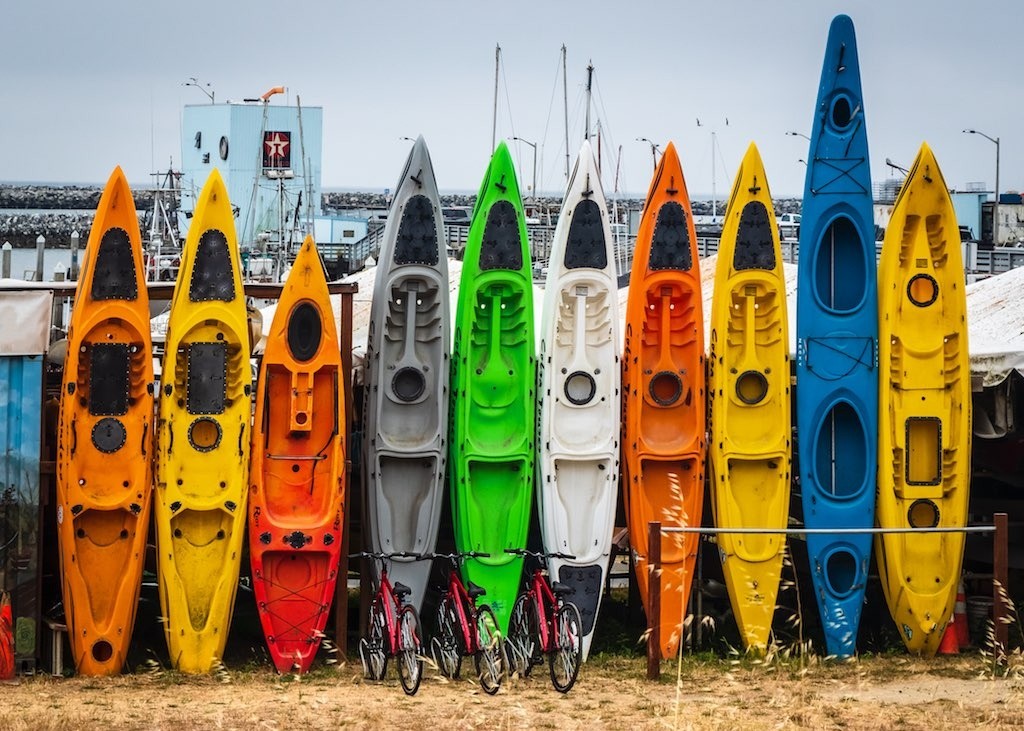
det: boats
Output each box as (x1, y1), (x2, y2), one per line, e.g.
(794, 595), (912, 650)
(450, 137), (536, 654)
(537, 137), (624, 666)
(154, 165), (248, 679)
(795, 12), (880, 664)
(622, 140), (708, 668)
(250, 234), (346, 681)
(54, 163), (156, 676)
(875, 140), (975, 657)
(359, 133), (451, 649)
(708, 139), (792, 662)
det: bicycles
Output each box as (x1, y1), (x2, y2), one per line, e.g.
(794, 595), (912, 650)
(504, 547), (583, 693)
(356, 550), (426, 696)
(416, 549), (506, 695)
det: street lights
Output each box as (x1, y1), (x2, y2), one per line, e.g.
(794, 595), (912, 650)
(180, 83), (214, 105)
(962, 129), (1000, 248)
(513, 137), (537, 199)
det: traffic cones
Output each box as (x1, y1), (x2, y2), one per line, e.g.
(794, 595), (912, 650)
(953, 577), (979, 653)
(938, 612), (963, 657)
